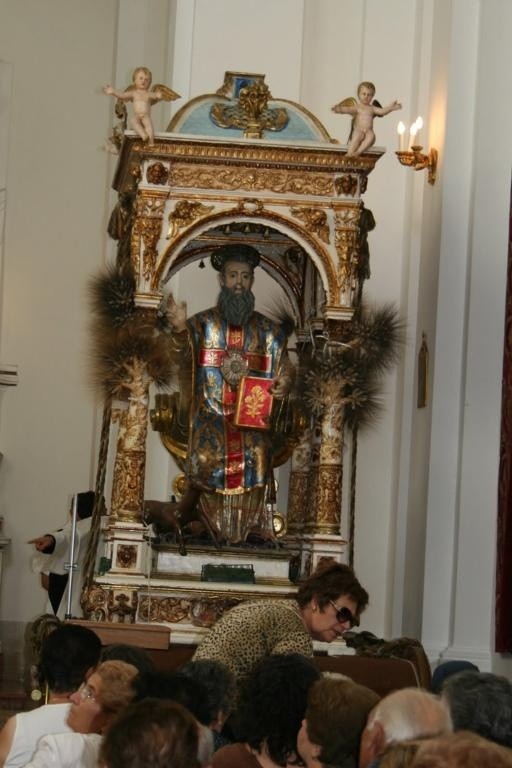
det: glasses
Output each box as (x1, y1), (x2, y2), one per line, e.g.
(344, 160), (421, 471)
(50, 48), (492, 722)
(328, 599), (360, 628)
(78, 683), (97, 703)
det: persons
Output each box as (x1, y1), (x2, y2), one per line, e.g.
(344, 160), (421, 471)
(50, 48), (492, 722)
(164, 244), (311, 548)
(297, 631), (512, 767)
(98, 64), (167, 149)
(28, 492), (109, 616)
(330, 79), (405, 161)
(0, 624), (324, 767)
(191, 561), (371, 752)
